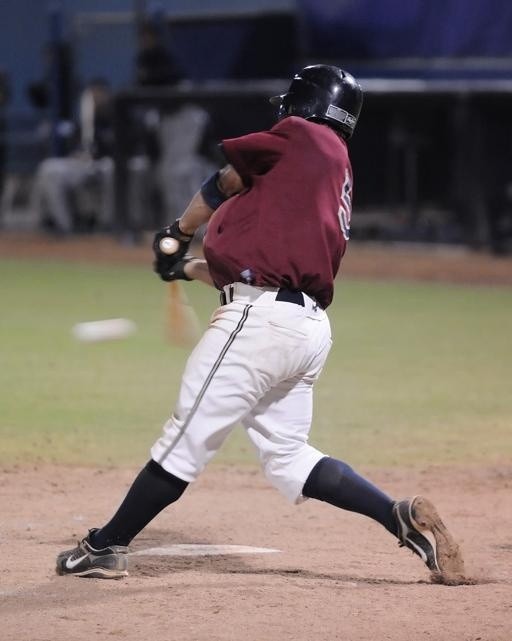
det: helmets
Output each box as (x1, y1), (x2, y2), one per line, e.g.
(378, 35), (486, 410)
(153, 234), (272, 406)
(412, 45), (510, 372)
(269, 64), (363, 141)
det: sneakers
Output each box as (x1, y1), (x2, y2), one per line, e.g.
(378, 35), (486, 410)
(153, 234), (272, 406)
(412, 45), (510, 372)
(56, 527), (128, 578)
(394, 495), (465, 581)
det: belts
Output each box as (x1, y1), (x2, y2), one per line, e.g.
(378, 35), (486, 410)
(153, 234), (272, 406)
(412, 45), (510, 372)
(219, 286), (304, 307)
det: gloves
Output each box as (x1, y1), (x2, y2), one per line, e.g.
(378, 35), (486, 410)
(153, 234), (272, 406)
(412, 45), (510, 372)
(155, 254), (198, 281)
(153, 220), (194, 270)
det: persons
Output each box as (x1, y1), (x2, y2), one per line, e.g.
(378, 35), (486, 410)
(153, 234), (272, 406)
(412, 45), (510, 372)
(55, 64), (466, 587)
